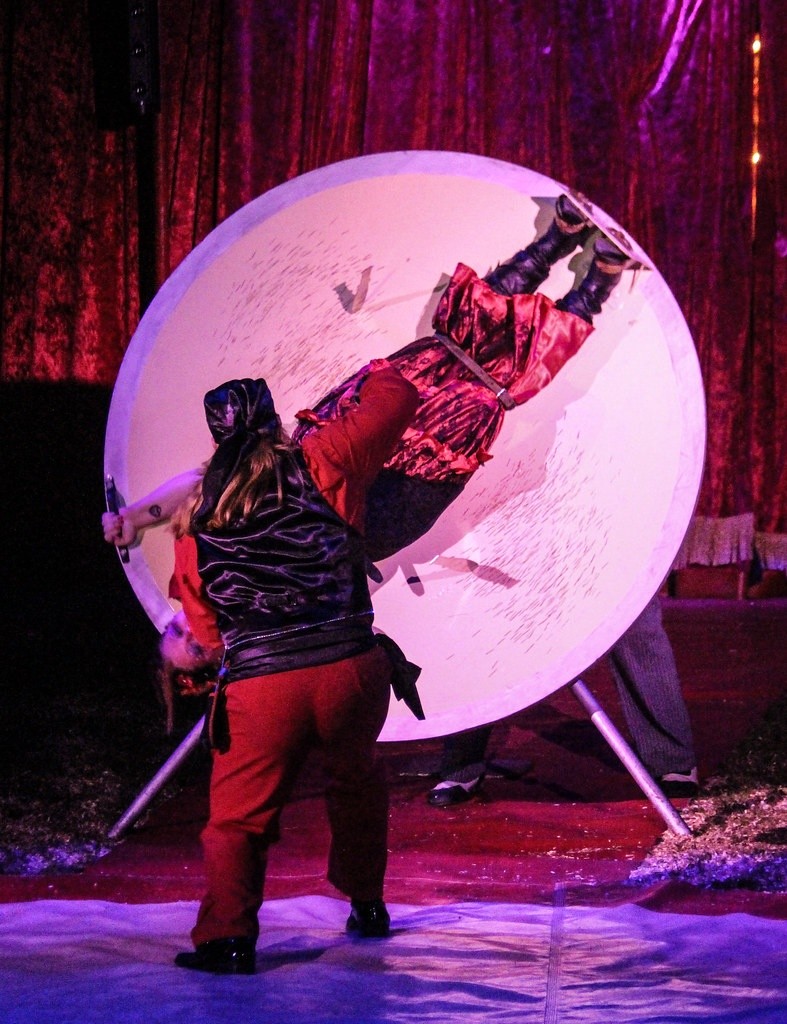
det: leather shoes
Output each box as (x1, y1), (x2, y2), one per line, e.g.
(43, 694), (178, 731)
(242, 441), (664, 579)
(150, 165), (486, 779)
(175, 937), (257, 974)
(346, 898), (390, 937)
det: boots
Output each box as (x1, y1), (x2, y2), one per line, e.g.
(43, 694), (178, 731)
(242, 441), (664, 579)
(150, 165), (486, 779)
(579, 227), (632, 303)
(526, 193), (595, 266)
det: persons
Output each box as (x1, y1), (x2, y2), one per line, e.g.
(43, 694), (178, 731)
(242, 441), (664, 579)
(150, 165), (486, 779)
(100, 192), (638, 733)
(428, 595), (699, 807)
(178, 357), (423, 970)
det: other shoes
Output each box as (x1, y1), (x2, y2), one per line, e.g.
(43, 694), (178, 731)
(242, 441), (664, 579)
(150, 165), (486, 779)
(428, 770), (484, 806)
(661, 767), (699, 797)
